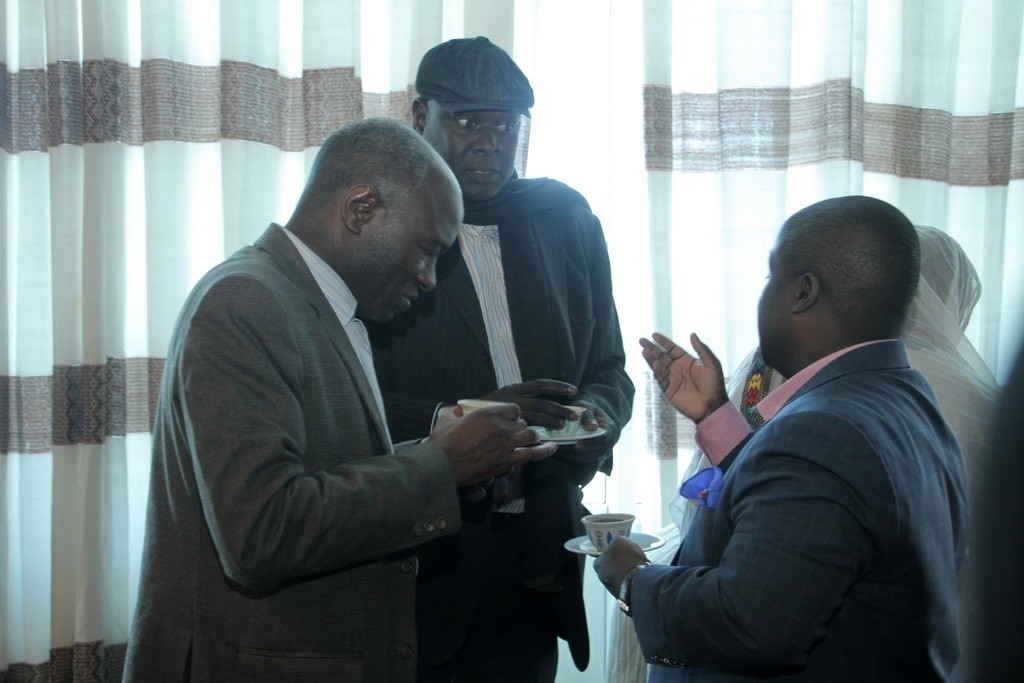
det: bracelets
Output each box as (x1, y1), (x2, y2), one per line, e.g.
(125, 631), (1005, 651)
(616, 562), (650, 616)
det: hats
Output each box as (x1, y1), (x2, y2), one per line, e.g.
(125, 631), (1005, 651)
(415, 36), (534, 118)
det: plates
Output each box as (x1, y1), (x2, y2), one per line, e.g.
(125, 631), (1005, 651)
(564, 533), (666, 557)
(526, 425), (606, 445)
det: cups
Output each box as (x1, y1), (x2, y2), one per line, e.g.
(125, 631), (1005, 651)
(581, 514), (635, 551)
(458, 400), (505, 417)
(545, 406), (587, 437)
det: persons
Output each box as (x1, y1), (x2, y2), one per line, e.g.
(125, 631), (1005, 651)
(141, 117), (558, 682)
(358, 32), (636, 683)
(589, 195), (997, 682)
